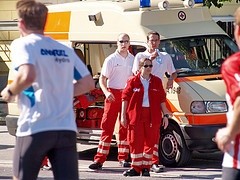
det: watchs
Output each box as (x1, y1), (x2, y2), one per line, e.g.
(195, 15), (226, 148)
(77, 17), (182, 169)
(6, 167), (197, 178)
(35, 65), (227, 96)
(163, 114), (169, 117)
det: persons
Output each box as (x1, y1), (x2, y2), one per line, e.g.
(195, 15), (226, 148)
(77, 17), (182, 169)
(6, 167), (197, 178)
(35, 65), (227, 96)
(183, 46), (209, 70)
(120, 58), (169, 176)
(214, 6), (240, 180)
(132, 31), (178, 173)
(40, 154), (50, 168)
(0, 0), (96, 180)
(88, 33), (136, 170)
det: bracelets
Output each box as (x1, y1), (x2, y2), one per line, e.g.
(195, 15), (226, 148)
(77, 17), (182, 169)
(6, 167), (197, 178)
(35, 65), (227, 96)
(7, 87), (15, 97)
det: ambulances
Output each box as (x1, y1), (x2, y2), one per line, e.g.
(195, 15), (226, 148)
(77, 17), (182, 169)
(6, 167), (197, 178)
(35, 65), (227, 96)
(5, 0), (240, 168)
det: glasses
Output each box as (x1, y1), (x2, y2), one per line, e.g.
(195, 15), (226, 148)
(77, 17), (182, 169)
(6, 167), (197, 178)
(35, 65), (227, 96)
(118, 40), (129, 43)
(143, 65), (153, 67)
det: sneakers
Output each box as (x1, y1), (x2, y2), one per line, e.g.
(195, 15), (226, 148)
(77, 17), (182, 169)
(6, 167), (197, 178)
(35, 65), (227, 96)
(150, 164), (161, 172)
(118, 160), (131, 168)
(142, 168), (150, 176)
(89, 161), (102, 169)
(41, 166), (51, 170)
(123, 167), (140, 176)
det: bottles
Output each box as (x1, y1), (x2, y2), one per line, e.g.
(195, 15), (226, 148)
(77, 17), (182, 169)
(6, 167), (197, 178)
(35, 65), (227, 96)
(155, 48), (163, 65)
